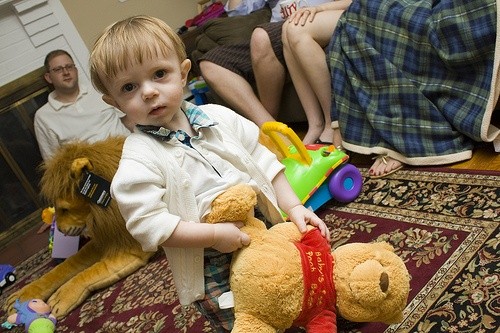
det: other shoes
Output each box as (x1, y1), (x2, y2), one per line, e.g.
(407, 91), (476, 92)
(314, 138), (332, 145)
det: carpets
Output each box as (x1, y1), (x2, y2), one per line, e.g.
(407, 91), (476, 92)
(0, 157), (500, 333)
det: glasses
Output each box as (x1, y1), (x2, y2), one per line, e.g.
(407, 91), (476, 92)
(48, 64), (75, 73)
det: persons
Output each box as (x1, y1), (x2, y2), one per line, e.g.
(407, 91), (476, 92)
(90, 14), (331, 332)
(33, 0), (500, 179)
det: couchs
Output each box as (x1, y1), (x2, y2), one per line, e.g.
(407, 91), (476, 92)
(191, 6), (308, 124)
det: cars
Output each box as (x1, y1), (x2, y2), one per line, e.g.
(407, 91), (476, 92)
(260, 120), (363, 224)
(0, 264), (17, 291)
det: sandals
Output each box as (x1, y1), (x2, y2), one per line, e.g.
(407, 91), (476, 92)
(369, 153), (405, 178)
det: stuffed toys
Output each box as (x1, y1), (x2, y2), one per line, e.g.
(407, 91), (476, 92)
(206, 184), (411, 333)
(0, 135), (156, 333)
